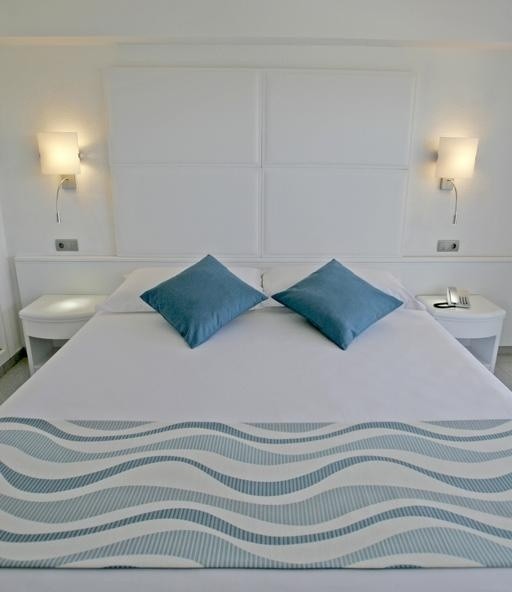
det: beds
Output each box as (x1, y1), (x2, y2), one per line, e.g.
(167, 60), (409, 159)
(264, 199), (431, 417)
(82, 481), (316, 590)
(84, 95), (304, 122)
(1, 267), (512, 592)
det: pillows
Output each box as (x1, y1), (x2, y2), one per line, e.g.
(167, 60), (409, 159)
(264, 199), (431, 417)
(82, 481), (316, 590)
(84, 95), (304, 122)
(139, 254), (269, 349)
(270, 258), (404, 351)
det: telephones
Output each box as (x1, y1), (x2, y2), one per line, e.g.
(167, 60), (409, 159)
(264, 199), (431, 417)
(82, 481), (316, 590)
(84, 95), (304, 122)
(447, 286), (472, 308)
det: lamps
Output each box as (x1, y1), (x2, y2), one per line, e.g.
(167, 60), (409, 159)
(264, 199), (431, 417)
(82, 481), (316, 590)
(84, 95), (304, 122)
(38, 131), (85, 223)
(437, 135), (478, 224)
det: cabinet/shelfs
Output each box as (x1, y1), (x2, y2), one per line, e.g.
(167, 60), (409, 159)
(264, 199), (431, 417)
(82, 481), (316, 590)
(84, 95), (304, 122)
(18, 293), (109, 377)
(417, 295), (505, 374)
